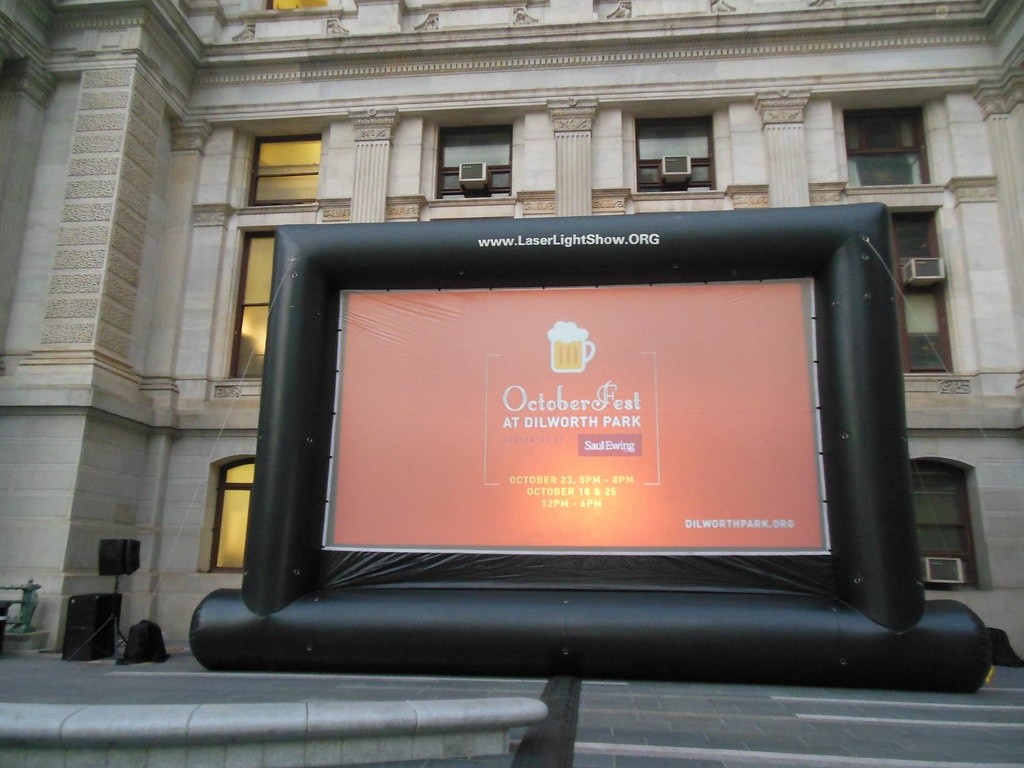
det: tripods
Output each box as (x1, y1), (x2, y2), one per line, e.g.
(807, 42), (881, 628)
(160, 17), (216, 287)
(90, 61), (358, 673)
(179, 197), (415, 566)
(68, 574), (127, 664)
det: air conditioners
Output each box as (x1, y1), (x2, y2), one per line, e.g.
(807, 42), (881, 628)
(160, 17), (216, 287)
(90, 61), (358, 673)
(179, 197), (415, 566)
(923, 557), (965, 584)
(659, 155), (692, 184)
(902, 258), (945, 286)
(459, 162), (493, 190)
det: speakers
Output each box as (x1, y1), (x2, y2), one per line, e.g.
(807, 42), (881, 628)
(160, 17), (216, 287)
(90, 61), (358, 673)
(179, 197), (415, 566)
(98, 539), (140, 577)
(63, 592), (123, 662)
